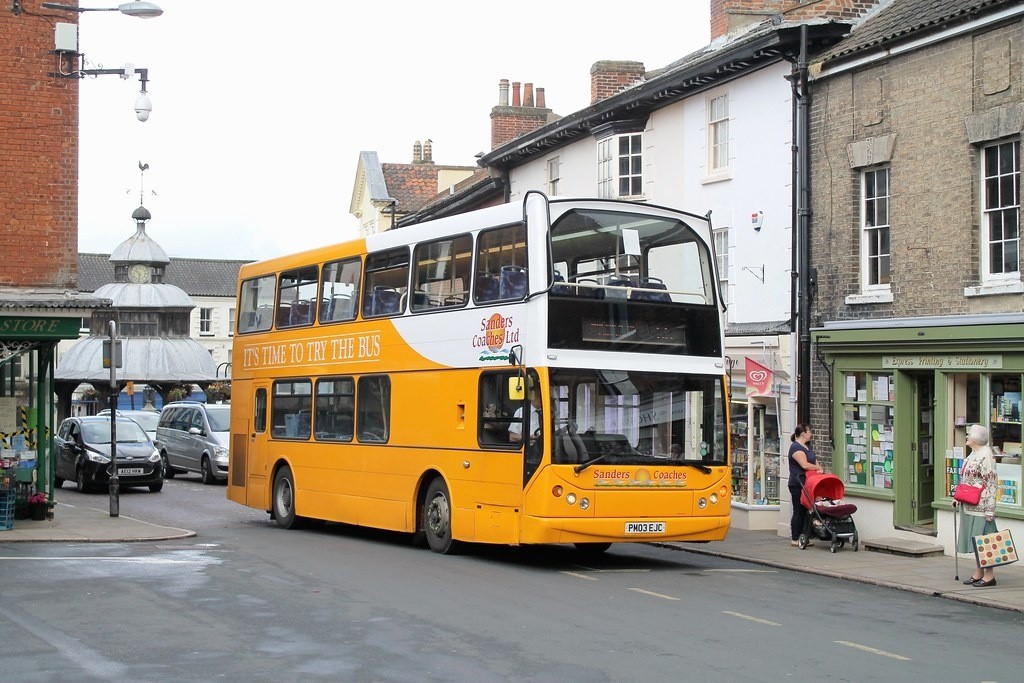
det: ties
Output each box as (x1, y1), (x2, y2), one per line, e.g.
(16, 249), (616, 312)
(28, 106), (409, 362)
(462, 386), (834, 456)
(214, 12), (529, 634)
(535, 410), (543, 432)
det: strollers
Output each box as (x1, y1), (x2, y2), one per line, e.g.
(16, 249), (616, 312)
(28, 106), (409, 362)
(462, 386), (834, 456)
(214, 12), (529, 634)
(794, 471), (860, 554)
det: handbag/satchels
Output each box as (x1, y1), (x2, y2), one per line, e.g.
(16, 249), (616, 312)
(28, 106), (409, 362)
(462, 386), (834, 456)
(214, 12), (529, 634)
(972, 529), (1019, 569)
(954, 484), (982, 506)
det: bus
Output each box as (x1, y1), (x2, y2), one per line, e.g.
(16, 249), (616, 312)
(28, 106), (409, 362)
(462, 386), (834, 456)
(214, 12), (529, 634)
(226, 189), (731, 555)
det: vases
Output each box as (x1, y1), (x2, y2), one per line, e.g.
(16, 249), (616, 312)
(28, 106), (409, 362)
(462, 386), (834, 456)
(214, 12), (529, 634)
(30, 503), (47, 520)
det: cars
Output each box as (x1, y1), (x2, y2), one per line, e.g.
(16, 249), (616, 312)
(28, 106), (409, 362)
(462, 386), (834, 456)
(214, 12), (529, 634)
(46, 416), (164, 492)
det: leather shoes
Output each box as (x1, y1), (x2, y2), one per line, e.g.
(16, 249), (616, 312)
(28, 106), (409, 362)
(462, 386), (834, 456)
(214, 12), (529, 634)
(963, 577), (981, 584)
(972, 576), (997, 587)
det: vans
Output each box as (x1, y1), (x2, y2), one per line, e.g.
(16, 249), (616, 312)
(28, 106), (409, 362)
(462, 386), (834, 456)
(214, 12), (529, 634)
(153, 400), (232, 484)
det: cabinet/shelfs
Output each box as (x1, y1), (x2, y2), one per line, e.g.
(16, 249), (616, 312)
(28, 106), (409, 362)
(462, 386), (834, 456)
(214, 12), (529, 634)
(0, 488), (16, 531)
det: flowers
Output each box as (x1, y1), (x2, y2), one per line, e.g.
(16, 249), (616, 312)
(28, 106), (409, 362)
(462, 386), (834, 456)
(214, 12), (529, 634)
(28, 492), (48, 503)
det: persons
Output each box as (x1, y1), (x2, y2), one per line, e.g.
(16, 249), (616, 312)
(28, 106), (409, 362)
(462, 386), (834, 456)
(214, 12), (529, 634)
(506, 383), (559, 445)
(950, 422), (1001, 588)
(787, 423), (826, 550)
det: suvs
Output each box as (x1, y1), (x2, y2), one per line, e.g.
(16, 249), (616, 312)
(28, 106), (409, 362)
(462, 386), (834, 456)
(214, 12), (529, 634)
(97, 407), (163, 447)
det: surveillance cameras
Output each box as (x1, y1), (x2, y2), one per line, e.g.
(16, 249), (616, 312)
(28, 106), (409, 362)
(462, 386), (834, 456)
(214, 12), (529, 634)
(134, 91), (152, 123)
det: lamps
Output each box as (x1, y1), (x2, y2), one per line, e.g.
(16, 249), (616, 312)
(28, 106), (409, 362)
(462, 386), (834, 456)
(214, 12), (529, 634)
(42, 0), (163, 20)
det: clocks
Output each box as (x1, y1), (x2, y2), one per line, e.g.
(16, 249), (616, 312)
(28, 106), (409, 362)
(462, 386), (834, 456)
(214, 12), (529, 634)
(128, 264), (150, 284)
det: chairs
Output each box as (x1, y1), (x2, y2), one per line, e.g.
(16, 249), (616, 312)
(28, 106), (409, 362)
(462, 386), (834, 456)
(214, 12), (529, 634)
(640, 276), (672, 304)
(270, 406), (389, 442)
(548, 270), (567, 295)
(577, 278), (602, 298)
(278, 302), (293, 326)
(372, 285), (406, 315)
(475, 271), (500, 303)
(326, 293), (353, 321)
(500, 266), (528, 299)
(350, 290), (375, 317)
(288, 300), (309, 325)
(607, 275), (639, 300)
(308, 296), (331, 323)
(255, 303), (274, 329)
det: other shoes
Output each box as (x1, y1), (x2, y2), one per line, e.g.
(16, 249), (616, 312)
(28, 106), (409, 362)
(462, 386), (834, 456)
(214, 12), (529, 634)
(791, 539), (814, 547)
